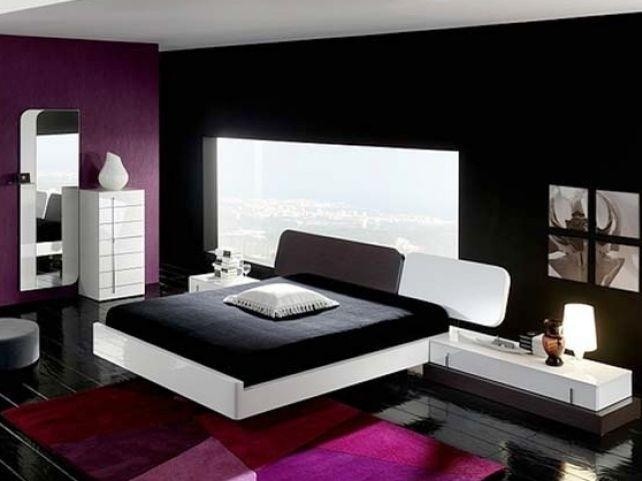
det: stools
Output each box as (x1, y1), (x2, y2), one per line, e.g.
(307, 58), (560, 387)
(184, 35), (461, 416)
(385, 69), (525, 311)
(0, 318), (40, 371)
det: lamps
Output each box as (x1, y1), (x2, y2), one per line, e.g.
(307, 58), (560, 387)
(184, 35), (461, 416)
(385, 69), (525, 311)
(561, 303), (597, 367)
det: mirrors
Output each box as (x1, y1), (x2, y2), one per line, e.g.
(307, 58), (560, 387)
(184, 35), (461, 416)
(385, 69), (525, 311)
(20, 109), (79, 292)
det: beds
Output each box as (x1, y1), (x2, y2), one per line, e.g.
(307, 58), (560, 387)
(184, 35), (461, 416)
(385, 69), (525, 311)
(92, 228), (512, 421)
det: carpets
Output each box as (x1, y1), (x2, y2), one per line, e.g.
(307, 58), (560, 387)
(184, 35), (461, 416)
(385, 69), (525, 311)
(1, 378), (511, 481)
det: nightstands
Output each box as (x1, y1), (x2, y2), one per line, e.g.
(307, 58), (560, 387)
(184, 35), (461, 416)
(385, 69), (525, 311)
(422, 329), (641, 448)
(188, 271), (260, 293)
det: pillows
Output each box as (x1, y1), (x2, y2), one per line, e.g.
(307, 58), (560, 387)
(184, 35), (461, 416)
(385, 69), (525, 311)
(223, 283), (340, 318)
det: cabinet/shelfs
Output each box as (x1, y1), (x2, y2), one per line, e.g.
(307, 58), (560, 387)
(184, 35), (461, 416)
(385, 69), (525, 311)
(78, 188), (146, 302)
